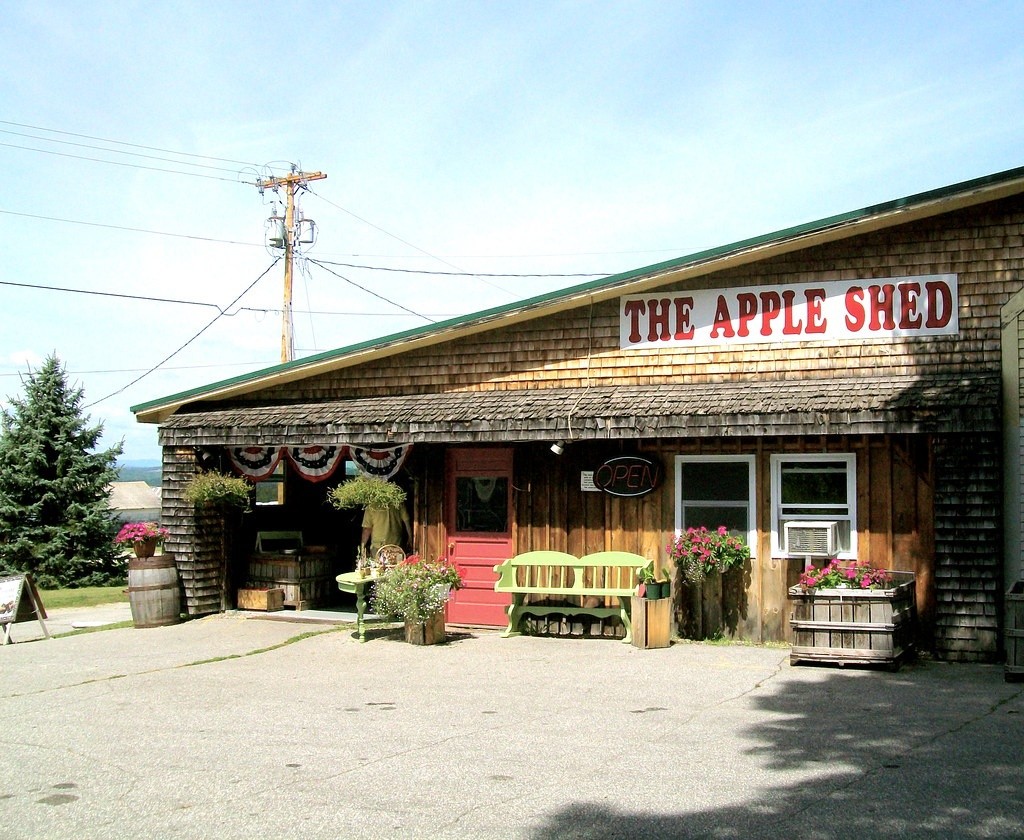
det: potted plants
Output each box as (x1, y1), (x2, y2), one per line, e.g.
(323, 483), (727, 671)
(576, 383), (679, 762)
(660, 568), (672, 598)
(179, 466), (256, 517)
(636, 560), (654, 599)
(642, 567), (661, 600)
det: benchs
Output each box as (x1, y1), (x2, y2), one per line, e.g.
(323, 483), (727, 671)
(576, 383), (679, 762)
(493, 550), (654, 644)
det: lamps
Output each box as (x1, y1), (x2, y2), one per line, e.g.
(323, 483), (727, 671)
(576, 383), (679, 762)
(550, 440), (565, 455)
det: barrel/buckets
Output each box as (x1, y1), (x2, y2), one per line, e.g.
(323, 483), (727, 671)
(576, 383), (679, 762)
(128, 554), (180, 628)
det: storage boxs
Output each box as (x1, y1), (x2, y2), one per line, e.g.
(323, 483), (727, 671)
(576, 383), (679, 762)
(237, 586), (285, 613)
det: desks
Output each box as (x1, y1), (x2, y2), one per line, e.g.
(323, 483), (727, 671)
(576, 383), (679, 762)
(336, 571), (391, 641)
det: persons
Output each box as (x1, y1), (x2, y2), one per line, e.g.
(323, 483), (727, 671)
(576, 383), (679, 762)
(360, 498), (412, 559)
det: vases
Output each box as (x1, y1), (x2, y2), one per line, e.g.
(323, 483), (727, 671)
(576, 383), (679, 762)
(788, 568), (917, 671)
(430, 582), (453, 601)
(135, 542), (157, 558)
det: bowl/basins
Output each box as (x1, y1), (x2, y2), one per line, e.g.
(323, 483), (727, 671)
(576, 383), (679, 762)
(279, 548), (297, 555)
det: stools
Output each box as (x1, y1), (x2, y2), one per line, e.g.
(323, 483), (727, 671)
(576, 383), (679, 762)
(630, 595), (673, 649)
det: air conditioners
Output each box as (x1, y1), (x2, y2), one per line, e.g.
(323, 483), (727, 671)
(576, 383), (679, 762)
(783, 521), (842, 556)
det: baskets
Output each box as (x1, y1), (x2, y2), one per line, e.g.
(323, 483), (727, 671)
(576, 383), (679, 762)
(375, 544), (406, 570)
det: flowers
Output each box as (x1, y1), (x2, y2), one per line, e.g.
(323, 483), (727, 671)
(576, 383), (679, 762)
(367, 554), (468, 626)
(799, 556), (894, 594)
(665, 526), (749, 585)
(113, 522), (171, 544)
